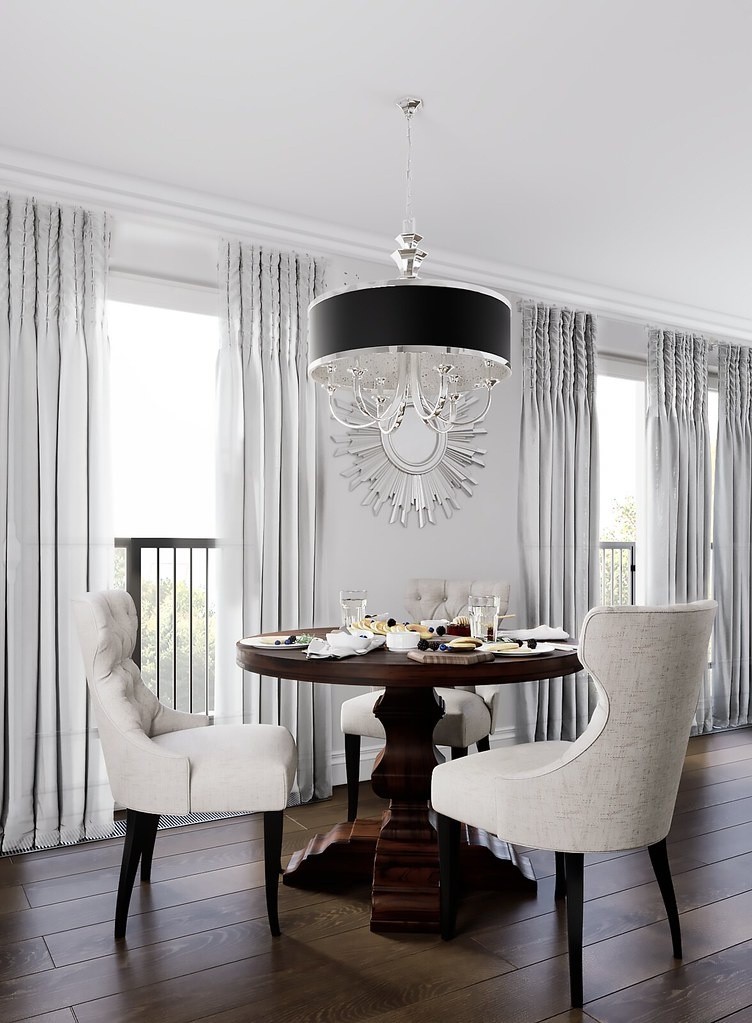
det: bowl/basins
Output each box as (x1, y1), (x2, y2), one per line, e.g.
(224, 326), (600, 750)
(421, 620), (447, 636)
(326, 630), (374, 650)
(386, 632), (420, 651)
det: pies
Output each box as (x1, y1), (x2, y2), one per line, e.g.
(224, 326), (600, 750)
(444, 637), (484, 651)
(349, 618), (433, 639)
(486, 643), (531, 652)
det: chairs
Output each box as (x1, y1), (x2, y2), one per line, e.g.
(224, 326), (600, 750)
(430, 596), (718, 1007)
(73, 588), (297, 941)
(339, 578), (512, 820)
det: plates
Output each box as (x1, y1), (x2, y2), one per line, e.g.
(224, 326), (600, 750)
(240, 636), (323, 649)
(475, 642), (554, 657)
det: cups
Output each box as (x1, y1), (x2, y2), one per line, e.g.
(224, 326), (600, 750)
(468, 595), (499, 643)
(340, 590), (367, 630)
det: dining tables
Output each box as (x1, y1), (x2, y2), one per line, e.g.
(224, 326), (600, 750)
(234, 627), (583, 934)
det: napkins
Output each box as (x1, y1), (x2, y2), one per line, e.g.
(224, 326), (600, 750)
(497, 625), (570, 640)
(302, 634), (386, 658)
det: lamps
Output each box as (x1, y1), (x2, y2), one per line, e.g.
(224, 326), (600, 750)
(306, 96), (513, 434)
